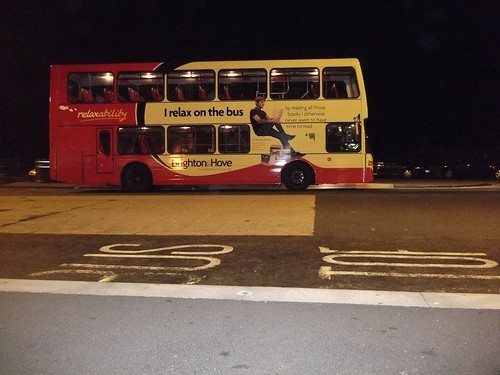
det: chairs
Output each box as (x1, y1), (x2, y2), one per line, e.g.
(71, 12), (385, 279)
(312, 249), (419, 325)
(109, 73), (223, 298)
(66, 81), (340, 154)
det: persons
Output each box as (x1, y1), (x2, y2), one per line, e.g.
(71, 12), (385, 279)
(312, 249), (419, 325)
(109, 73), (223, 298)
(328, 127), (349, 152)
(252, 98), (308, 158)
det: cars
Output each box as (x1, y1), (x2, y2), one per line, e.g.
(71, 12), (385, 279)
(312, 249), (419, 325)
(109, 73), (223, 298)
(371, 151), (433, 180)
(5, 157), (48, 179)
(433, 150), (500, 179)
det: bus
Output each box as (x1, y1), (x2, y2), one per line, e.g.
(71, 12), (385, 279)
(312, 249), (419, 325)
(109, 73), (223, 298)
(47, 57), (376, 194)
